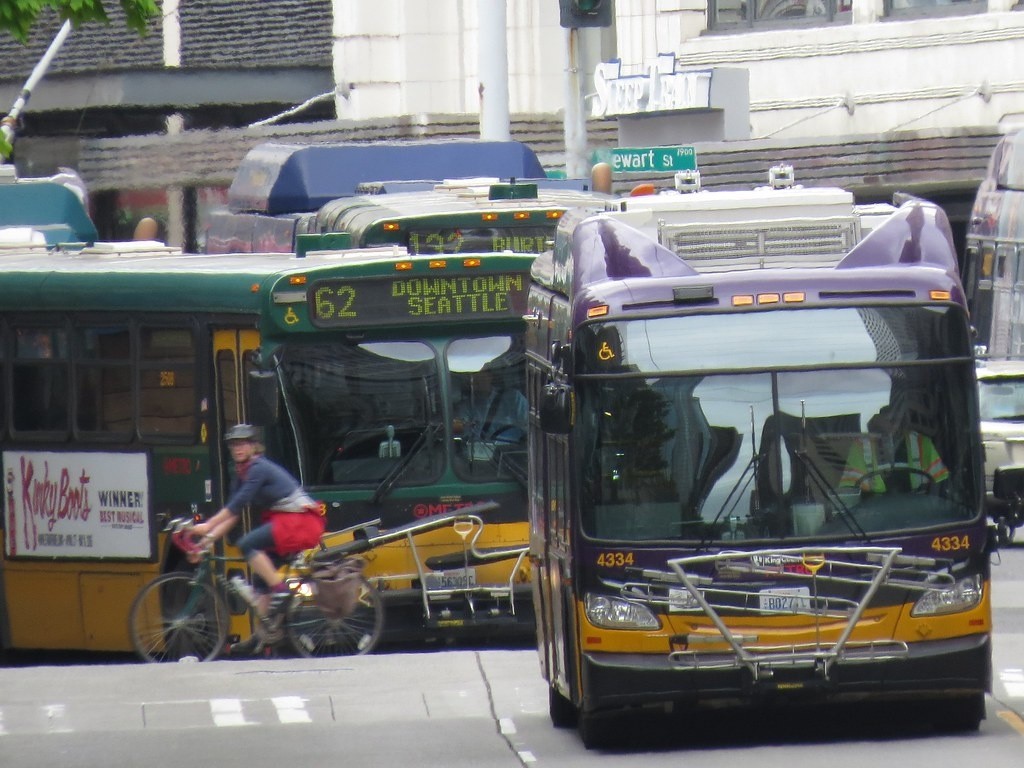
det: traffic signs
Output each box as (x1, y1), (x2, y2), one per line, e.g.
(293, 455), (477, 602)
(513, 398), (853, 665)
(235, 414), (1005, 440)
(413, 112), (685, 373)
(586, 146), (697, 177)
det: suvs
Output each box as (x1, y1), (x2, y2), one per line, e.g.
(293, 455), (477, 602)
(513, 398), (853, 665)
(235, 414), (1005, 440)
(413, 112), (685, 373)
(976, 360), (1024, 533)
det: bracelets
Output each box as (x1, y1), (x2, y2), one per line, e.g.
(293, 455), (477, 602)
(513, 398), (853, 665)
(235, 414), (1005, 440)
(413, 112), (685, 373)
(206, 533), (215, 539)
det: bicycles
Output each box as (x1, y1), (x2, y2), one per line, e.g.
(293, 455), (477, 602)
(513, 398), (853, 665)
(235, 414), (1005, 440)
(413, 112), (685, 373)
(127, 514), (382, 660)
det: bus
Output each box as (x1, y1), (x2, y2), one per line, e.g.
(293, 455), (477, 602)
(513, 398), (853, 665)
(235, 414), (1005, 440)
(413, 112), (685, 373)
(967, 123), (1024, 360)
(1, 241), (546, 660)
(298, 179), (618, 252)
(202, 141), (547, 249)
(523, 184), (989, 749)
(1, 166), (98, 242)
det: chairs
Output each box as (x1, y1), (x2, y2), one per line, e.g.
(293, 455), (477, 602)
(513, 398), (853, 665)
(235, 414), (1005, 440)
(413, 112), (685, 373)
(808, 431), (868, 504)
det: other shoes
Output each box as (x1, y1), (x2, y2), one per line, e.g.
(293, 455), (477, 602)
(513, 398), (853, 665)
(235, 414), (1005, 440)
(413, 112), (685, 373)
(268, 589), (294, 615)
(230, 634), (258, 653)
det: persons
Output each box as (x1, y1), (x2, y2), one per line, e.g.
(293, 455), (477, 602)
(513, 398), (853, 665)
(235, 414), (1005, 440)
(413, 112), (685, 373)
(837, 408), (950, 506)
(192, 424), (324, 622)
(458, 371), (528, 444)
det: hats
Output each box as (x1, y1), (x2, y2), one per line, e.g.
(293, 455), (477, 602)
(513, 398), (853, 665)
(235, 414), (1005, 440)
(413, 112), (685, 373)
(223, 424), (260, 441)
